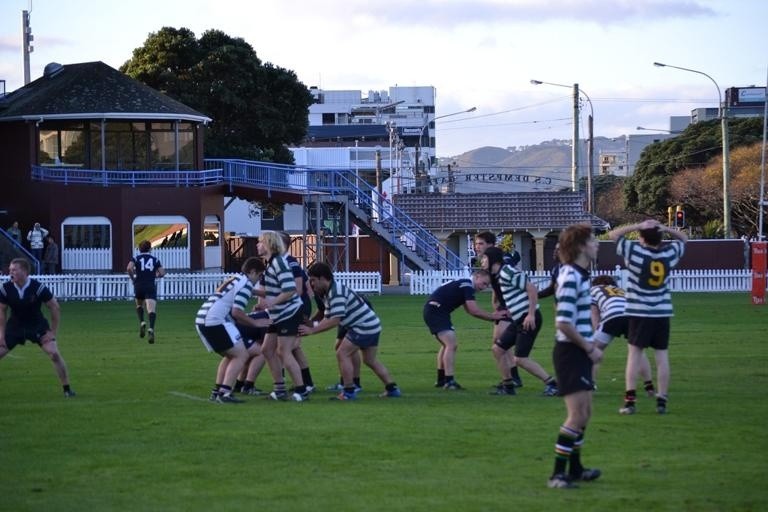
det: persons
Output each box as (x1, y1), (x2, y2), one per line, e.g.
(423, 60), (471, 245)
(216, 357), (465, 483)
(537, 240), (563, 303)
(195, 230), (402, 404)
(127, 240), (165, 344)
(5, 223), (59, 274)
(547, 223), (604, 487)
(425, 269), (511, 390)
(608, 220), (688, 416)
(468, 232), (558, 396)
(590, 276), (656, 397)
(0, 258), (76, 398)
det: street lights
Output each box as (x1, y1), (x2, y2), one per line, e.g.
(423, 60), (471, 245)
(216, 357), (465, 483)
(635, 125), (683, 136)
(418, 107), (476, 169)
(653, 61), (733, 238)
(529, 79), (596, 217)
(386, 119), (407, 218)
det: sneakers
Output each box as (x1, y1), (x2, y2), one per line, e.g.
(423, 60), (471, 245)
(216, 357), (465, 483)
(325, 383), (401, 400)
(547, 468), (602, 490)
(147, 327), (154, 344)
(435, 376), (466, 392)
(63, 390), (77, 398)
(488, 376), (562, 397)
(209, 382), (317, 403)
(618, 389), (669, 415)
(139, 321), (147, 337)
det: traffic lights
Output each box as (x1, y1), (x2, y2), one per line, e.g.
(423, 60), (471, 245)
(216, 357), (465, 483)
(675, 210), (685, 228)
